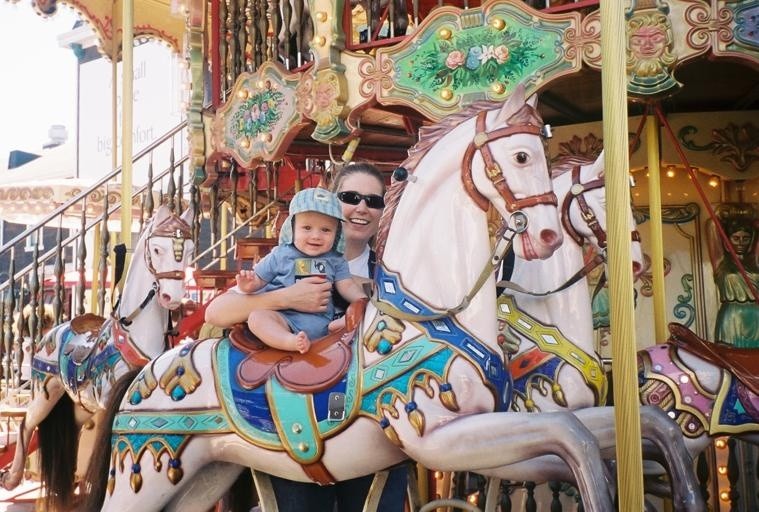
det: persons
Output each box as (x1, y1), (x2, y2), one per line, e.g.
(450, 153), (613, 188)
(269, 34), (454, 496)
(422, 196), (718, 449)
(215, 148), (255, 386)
(237, 186), (369, 353)
(205, 163), (409, 512)
(703, 202), (759, 350)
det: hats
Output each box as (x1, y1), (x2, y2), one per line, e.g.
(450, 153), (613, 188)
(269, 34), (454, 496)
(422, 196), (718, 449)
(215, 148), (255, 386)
(280, 187), (350, 254)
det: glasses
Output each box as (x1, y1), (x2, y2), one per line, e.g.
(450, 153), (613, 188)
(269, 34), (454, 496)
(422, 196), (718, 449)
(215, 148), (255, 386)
(336, 191), (385, 209)
